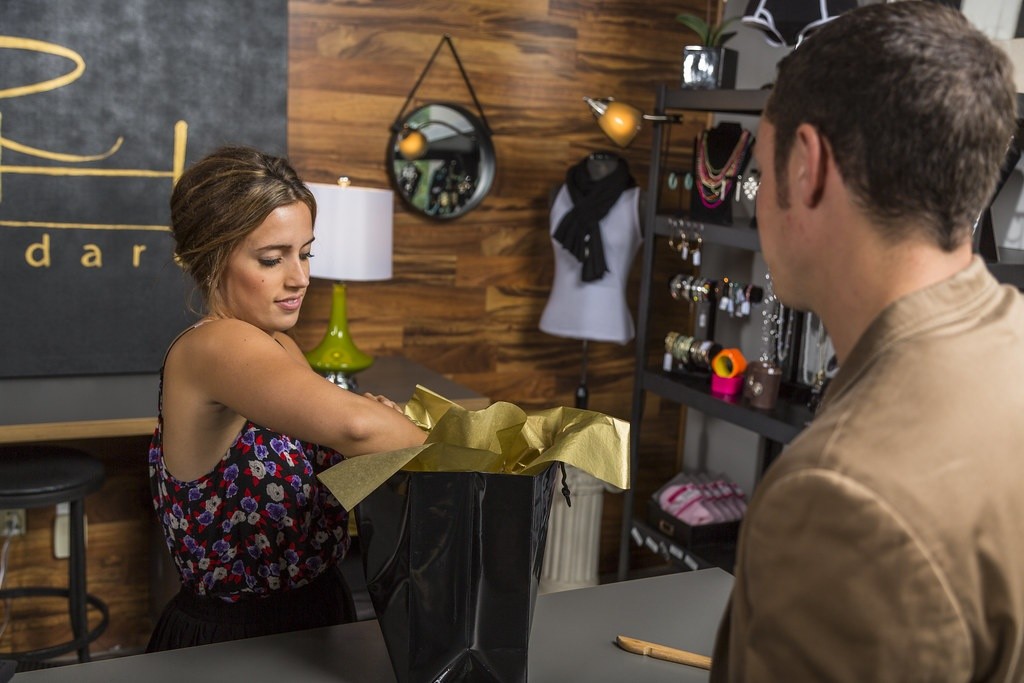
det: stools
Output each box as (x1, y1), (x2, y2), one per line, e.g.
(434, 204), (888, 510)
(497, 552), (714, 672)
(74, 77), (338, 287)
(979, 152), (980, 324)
(0, 446), (113, 677)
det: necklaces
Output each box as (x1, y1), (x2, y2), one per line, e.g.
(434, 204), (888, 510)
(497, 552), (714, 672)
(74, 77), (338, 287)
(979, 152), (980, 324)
(693, 126), (757, 209)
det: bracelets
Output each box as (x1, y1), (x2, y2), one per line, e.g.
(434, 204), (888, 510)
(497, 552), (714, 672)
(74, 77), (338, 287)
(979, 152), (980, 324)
(662, 329), (781, 411)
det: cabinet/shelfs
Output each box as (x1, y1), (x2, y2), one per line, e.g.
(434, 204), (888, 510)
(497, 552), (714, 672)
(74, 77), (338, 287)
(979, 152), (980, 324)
(614, 78), (1023, 592)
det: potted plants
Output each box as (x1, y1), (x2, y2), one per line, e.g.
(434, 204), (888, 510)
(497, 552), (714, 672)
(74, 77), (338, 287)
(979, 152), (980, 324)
(677, 12), (743, 90)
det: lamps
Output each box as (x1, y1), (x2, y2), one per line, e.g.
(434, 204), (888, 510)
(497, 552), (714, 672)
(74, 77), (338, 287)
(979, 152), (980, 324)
(301, 176), (395, 372)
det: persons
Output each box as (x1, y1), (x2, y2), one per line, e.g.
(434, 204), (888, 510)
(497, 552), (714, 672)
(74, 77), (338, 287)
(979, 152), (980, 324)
(146, 144), (440, 658)
(705, 0), (1023, 680)
(535, 151), (652, 347)
